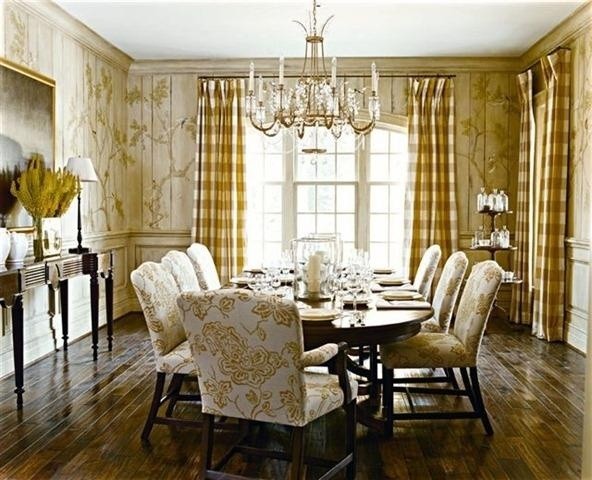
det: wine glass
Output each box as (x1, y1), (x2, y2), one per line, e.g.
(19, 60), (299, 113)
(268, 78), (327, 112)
(328, 249), (375, 319)
(248, 250), (293, 297)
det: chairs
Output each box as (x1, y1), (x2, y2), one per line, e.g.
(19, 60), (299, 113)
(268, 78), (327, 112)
(161, 249), (201, 394)
(130, 261), (253, 442)
(371, 250), (470, 399)
(378, 260), (504, 437)
(177, 288), (359, 480)
(360, 245), (442, 376)
(186, 242), (222, 290)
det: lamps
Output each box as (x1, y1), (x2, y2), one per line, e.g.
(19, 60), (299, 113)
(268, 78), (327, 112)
(66, 156), (98, 254)
(239, 0), (380, 167)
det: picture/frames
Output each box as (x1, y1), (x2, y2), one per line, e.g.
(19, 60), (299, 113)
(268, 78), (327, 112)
(0, 57), (58, 234)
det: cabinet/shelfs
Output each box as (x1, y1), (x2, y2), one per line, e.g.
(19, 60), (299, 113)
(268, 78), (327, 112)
(466, 210), (524, 335)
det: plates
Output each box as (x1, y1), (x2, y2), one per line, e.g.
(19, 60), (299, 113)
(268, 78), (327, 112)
(244, 268), (265, 274)
(299, 308), (339, 323)
(230, 277), (252, 285)
(374, 267), (421, 301)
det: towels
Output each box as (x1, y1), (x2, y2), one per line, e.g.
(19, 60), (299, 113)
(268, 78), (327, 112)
(376, 300), (431, 309)
(372, 273), (393, 279)
(371, 284), (417, 292)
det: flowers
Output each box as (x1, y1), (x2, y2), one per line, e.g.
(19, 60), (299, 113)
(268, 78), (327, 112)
(8, 153), (77, 217)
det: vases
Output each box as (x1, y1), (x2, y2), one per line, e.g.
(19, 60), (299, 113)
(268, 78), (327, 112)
(32, 215), (43, 258)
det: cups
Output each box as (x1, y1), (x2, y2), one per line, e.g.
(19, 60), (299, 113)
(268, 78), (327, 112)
(504, 270), (514, 283)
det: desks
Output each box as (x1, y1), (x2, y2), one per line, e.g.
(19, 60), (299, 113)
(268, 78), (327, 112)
(0, 249), (115, 412)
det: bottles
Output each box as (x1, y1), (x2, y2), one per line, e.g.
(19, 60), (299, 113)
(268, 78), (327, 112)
(476, 187), (508, 211)
(471, 225), (510, 249)
